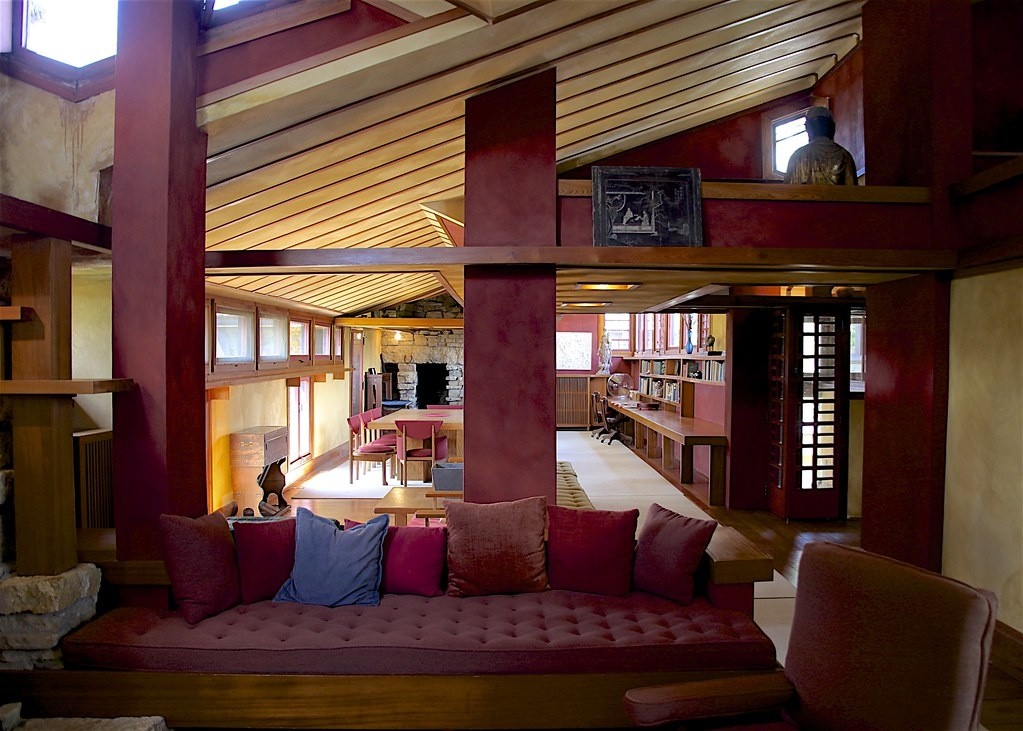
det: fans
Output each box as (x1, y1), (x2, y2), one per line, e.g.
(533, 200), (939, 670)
(607, 373), (635, 397)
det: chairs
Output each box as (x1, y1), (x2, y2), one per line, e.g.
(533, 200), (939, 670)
(590, 391), (633, 446)
(347, 404), (464, 487)
(623, 542), (996, 731)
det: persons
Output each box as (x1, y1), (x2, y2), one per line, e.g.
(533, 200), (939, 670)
(785, 106), (858, 188)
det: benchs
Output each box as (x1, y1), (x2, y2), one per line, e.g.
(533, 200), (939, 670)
(56, 523), (777, 731)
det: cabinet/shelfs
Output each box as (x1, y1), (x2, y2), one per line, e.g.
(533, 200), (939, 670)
(231, 426), (290, 521)
(623, 355), (727, 417)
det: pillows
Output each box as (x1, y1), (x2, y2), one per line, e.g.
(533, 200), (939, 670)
(158, 496), (718, 605)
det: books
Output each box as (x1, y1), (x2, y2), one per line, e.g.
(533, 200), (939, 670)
(639, 360), (725, 404)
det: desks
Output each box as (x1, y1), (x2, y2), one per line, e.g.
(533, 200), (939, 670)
(374, 488), (444, 527)
(606, 395), (727, 505)
(367, 409), (464, 480)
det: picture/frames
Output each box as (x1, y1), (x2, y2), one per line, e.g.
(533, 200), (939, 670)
(592, 166), (704, 248)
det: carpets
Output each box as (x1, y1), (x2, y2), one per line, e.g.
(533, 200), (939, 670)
(556, 459), (595, 508)
(292, 457), (432, 499)
